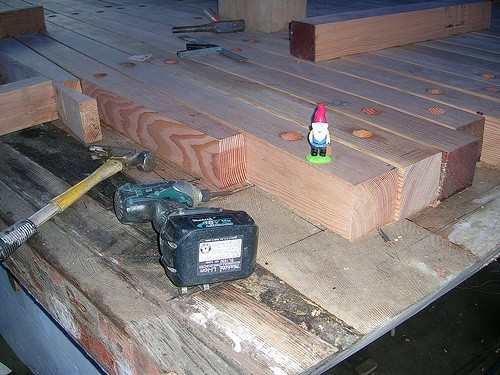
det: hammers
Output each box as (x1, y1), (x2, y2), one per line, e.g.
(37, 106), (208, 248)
(0, 145), (157, 266)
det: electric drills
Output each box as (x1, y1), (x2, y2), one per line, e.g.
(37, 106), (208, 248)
(113, 182), (235, 226)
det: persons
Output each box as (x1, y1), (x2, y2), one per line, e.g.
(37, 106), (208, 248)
(308, 103), (331, 157)
